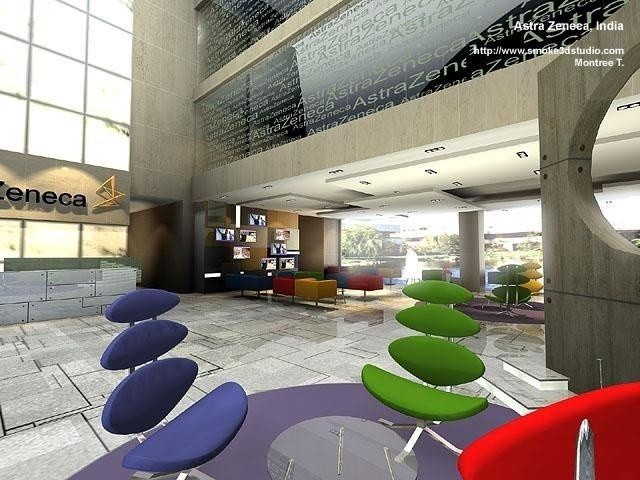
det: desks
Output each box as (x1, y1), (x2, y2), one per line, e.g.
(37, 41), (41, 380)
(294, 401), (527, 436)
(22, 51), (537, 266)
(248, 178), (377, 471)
(264, 415), (420, 479)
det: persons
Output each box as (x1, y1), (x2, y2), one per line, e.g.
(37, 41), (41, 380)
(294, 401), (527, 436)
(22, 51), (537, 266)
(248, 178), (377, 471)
(262, 260), (276, 270)
(234, 247), (250, 259)
(276, 231), (290, 240)
(274, 244), (286, 254)
(218, 229), (234, 240)
(240, 232), (256, 242)
(251, 214), (265, 225)
(282, 259), (294, 268)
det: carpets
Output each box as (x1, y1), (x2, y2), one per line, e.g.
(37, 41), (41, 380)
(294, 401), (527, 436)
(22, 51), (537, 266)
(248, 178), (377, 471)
(65, 384), (522, 480)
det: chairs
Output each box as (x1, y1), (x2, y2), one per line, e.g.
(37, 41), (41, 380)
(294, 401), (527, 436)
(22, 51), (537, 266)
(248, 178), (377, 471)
(362, 262), (640, 479)
(225, 270), (384, 307)
(100, 289), (249, 479)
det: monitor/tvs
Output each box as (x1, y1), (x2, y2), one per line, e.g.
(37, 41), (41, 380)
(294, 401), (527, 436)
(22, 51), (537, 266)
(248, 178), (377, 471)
(279, 257), (295, 270)
(232, 246), (251, 260)
(260, 258), (277, 270)
(248, 213), (267, 227)
(274, 228), (291, 241)
(239, 230), (257, 242)
(270, 242), (287, 256)
(215, 228), (235, 242)
